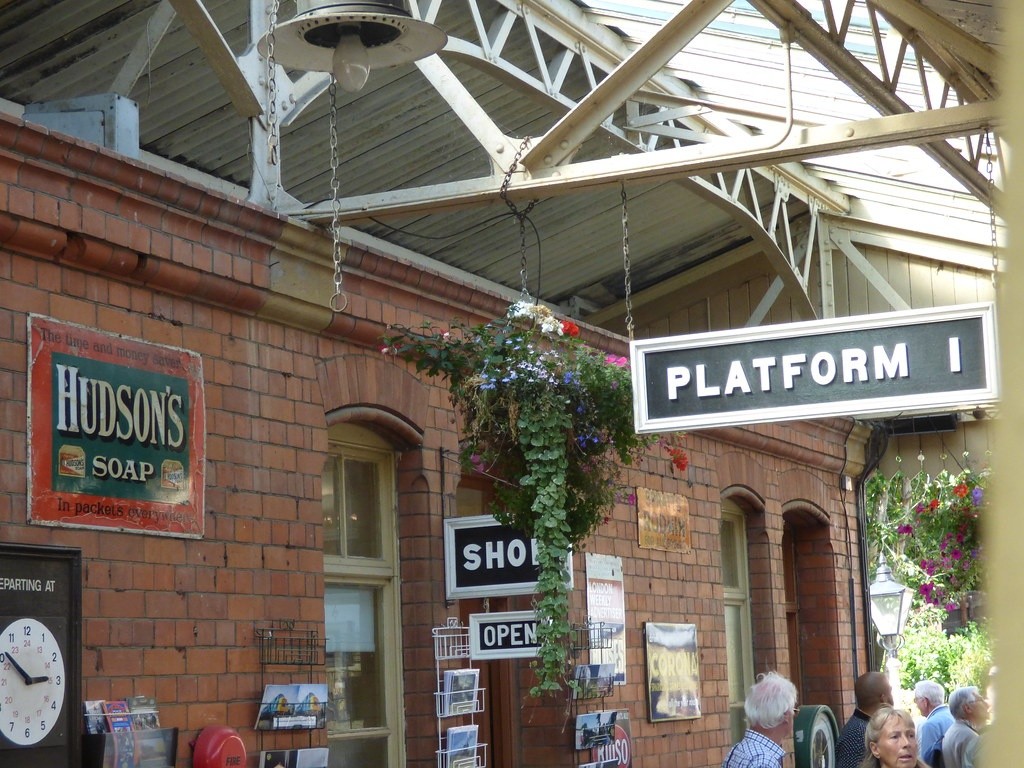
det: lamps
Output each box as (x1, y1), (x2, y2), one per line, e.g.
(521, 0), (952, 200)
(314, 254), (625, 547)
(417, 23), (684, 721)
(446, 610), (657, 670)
(256, 0), (448, 93)
(868, 552), (918, 657)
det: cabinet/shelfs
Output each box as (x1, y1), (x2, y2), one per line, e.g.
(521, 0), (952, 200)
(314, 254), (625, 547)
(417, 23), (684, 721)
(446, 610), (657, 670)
(431, 616), (490, 768)
(568, 615), (626, 768)
(255, 619), (330, 768)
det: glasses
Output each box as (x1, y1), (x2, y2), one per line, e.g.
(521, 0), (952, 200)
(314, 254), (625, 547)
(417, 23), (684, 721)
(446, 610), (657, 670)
(968, 697), (984, 703)
(790, 706), (800, 718)
(913, 697), (920, 703)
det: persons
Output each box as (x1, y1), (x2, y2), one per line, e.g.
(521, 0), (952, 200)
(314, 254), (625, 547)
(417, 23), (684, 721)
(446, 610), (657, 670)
(722, 671), (798, 768)
(837, 667), (997, 768)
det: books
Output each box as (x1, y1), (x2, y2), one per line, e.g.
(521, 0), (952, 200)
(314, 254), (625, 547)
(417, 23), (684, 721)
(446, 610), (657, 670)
(443, 668), (480, 768)
(259, 748), (329, 768)
(256, 684), (328, 730)
(574, 663), (618, 768)
(83, 695), (167, 768)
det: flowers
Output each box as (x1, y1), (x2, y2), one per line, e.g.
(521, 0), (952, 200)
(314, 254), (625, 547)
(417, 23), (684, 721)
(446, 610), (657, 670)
(377, 300), (690, 705)
(871, 485), (987, 611)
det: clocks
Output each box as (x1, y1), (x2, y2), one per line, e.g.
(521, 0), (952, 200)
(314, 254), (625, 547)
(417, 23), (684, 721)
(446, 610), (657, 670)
(793, 704), (839, 768)
(0, 543), (83, 768)
